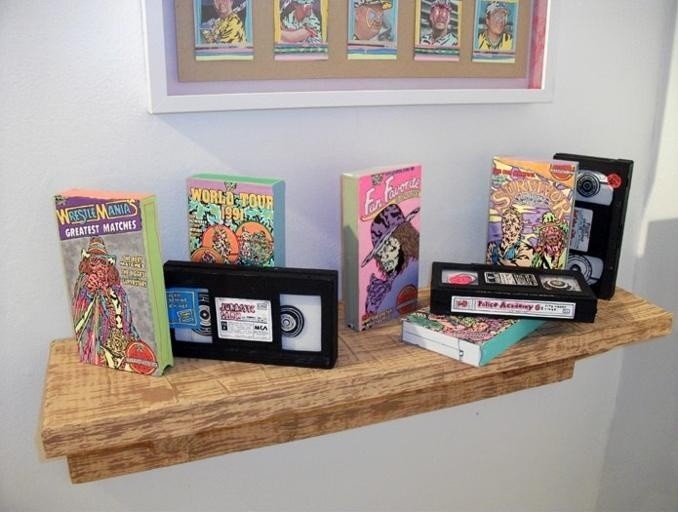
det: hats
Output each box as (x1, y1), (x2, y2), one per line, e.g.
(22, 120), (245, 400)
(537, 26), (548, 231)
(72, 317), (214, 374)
(293, 0), (314, 4)
(80, 236), (116, 267)
(360, 204), (420, 267)
(430, 0), (452, 10)
(539, 211), (558, 229)
(485, 1), (507, 14)
(354, 0), (393, 10)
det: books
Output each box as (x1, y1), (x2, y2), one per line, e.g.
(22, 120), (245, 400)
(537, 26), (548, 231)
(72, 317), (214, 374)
(485, 155), (579, 270)
(53, 188), (174, 375)
(339, 164), (422, 333)
(403, 314), (547, 366)
(188, 174), (287, 266)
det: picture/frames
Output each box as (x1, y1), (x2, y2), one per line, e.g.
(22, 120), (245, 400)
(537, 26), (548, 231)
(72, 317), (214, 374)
(140, 1), (557, 116)
